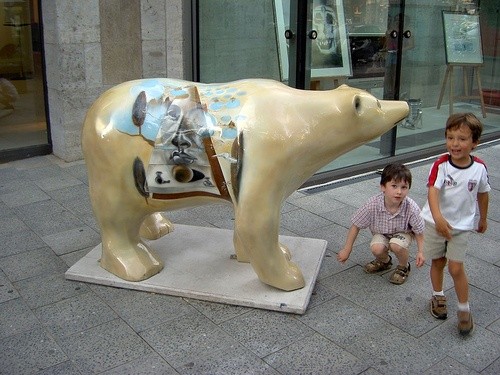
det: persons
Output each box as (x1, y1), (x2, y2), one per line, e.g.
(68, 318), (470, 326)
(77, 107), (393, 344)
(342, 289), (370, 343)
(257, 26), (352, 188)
(418, 113), (491, 336)
(337, 164), (425, 285)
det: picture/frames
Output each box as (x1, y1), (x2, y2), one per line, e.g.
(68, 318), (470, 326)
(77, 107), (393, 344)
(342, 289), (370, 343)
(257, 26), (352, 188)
(441, 9), (486, 67)
(271, 0), (353, 82)
(346, 32), (389, 83)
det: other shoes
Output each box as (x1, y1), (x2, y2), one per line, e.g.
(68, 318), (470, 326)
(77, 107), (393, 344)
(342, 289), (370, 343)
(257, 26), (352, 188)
(363, 255), (393, 273)
(431, 293), (448, 320)
(457, 310), (474, 335)
(389, 262), (411, 285)
